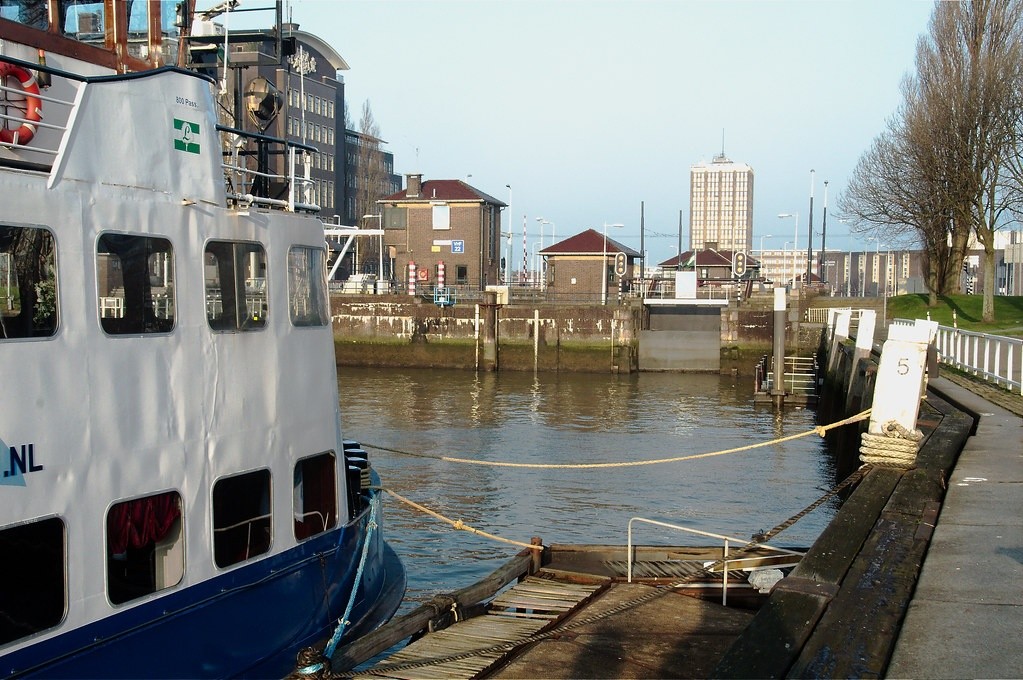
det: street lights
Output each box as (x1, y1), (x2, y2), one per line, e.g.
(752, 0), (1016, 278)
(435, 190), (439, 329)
(807, 168), (828, 295)
(868, 237), (890, 296)
(760, 214), (800, 289)
(535, 216), (556, 291)
(600, 222), (625, 305)
(504, 184), (513, 283)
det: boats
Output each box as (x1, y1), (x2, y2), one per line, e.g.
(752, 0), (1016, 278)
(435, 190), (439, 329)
(0, 0), (415, 680)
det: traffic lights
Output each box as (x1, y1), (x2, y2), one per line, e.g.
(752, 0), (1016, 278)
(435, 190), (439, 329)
(733, 252), (748, 279)
(615, 252), (628, 277)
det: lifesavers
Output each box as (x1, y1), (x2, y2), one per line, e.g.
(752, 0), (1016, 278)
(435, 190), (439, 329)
(0, 61), (43, 145)
